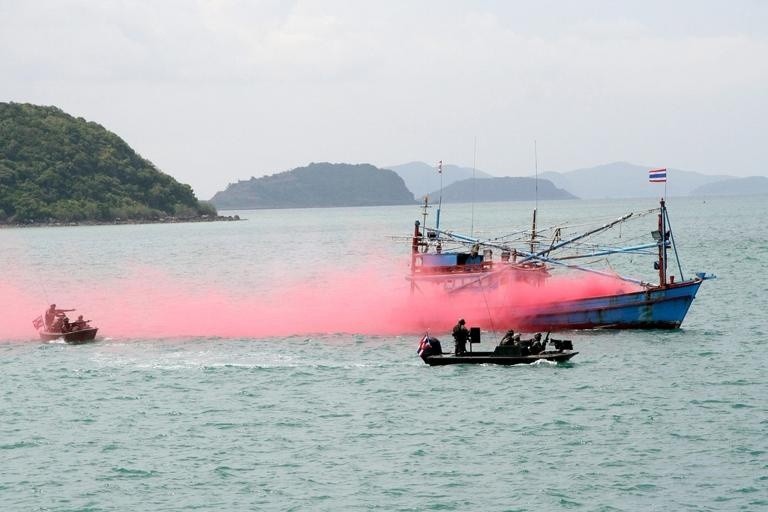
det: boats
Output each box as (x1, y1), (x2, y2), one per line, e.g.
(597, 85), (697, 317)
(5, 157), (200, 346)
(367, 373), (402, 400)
(427, 327), (579, 366)
(39, 323), (97, 344)
(405, 139), (706, 331)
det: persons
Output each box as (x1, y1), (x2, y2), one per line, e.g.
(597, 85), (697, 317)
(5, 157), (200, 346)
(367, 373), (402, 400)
(44, 303), (89, 335)
(529, 332), (549, 355)
(451, 318), (470, 356)
(498, 329), (514, 347)
(510, 333), (534, 356)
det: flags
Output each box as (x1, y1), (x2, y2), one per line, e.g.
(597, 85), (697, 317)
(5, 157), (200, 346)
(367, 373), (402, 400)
(647, 167), (666, 184)
(415, 334), (430, 357)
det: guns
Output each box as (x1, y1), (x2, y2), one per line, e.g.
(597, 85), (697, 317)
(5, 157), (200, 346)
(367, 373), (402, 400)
(66, 320), (91, 328)
(52, 308), (76, 314)
(541, 326), (553, 351)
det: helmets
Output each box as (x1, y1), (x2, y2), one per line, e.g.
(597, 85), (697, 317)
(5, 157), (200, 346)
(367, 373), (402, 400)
(506, 329), (514, 336)
(63, 317), (69, 322)
(51, 304), (56, 307)
(534, 332), (542, 340)
(513, 332), (520, 339)
(458, 319), (465, 325)
